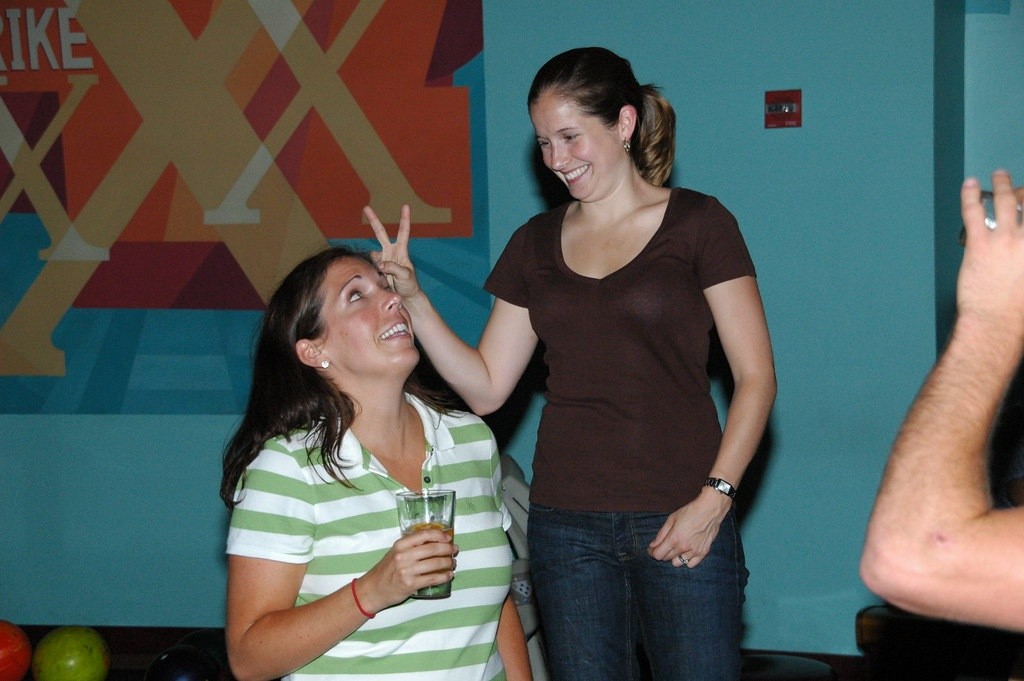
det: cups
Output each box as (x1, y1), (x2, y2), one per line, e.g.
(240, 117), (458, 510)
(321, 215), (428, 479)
(395, 489), (456, 600)
(958, 189), (1022, 246)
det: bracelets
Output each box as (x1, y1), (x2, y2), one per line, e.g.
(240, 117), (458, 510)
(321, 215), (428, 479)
(351, 577), (376, 620)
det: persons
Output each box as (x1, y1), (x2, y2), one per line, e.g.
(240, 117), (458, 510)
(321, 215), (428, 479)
(219, 249), (534, 681)
(358, 46), (777, 681)
(859, 166), (1023, 632)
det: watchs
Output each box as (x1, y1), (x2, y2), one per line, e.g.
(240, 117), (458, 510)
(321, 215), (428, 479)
(703, 478), (736, 503)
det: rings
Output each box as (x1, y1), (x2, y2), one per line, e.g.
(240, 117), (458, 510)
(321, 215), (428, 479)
(678, 555), (687, 563)
